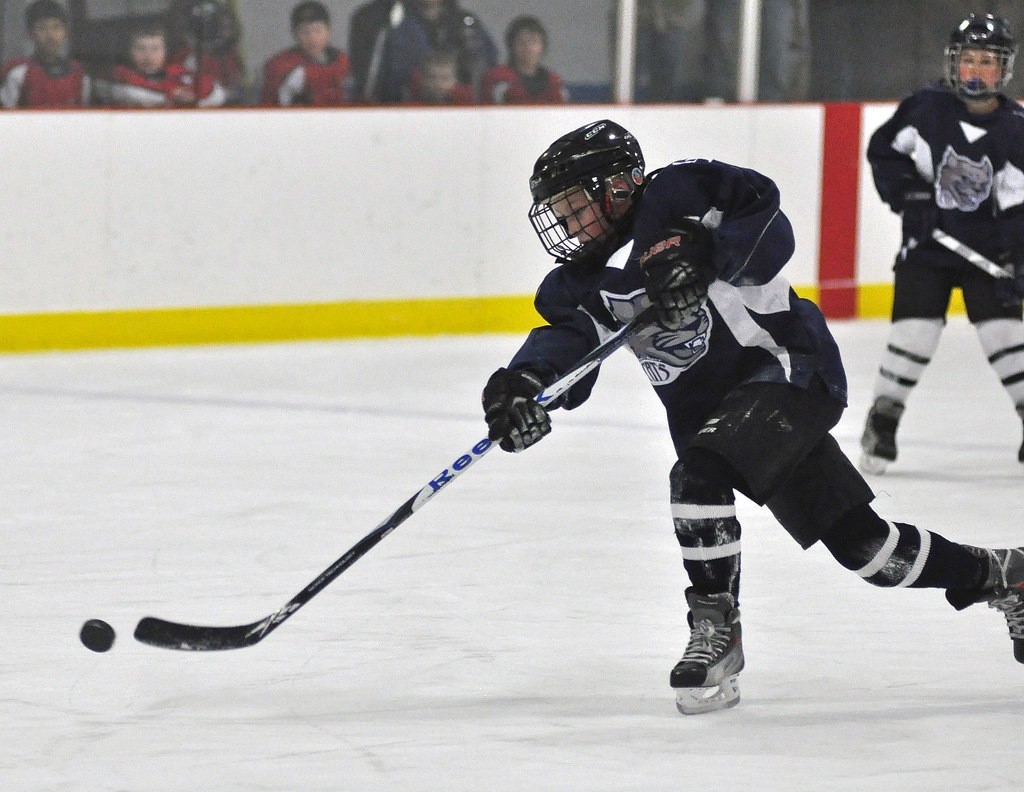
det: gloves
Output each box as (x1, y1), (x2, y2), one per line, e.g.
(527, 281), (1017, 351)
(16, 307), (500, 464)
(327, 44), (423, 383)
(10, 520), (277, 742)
(994, 253), (1024, 308)
(482, 368), (553, 453)
(643, 216), (716, 332)
(900, 187), (945, 251)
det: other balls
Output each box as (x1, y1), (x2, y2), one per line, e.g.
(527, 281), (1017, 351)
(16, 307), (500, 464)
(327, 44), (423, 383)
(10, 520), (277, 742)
(929, 227), (1015, 282)
(78, 618), (117, 654)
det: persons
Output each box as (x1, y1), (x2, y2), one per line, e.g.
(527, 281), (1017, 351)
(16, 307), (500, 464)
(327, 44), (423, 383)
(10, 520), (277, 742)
(439, 14), (571, 102)
(859, 12), (1024, 473)
(483, 119), (1024, 713)
(605, 0), (1024, 101)
(0, 1), (495, 103)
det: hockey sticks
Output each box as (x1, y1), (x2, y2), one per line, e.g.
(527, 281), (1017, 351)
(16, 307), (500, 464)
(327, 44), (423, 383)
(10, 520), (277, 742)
(132, 301), (663, 653)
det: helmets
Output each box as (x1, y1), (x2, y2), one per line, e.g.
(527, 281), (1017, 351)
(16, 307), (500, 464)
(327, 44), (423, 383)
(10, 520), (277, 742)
(943, 13), (1018, 101)
(528, 120), (646, 271)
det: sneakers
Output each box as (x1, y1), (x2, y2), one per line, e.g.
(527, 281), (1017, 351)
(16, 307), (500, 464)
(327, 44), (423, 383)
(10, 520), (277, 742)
(858, 397), (905, 474)
(944, 544), (1024, 662)
(670, 585), (745, 716)
